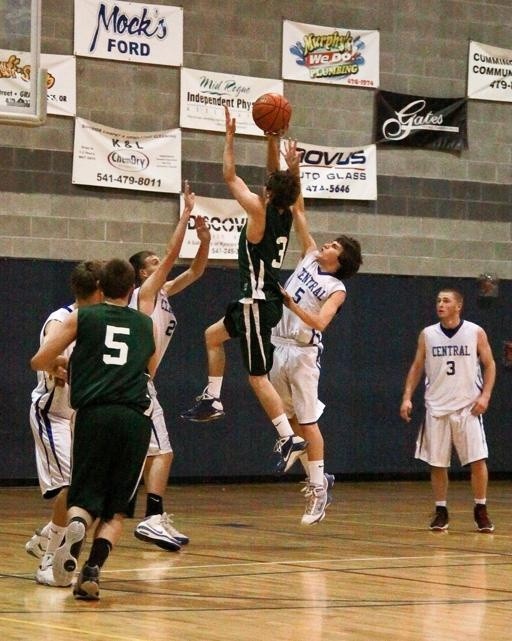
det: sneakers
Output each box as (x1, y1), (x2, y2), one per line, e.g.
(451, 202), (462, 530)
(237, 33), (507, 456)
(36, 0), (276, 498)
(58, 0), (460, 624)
(272, 433), (335, 526)
(25, 511), (189, 600)
(178, 393), (225, 423)
(473, 503), (494, 533)
(429, 506), (449, 531)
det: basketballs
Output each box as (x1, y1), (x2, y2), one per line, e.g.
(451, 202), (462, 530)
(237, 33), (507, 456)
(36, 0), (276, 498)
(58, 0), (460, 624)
(252, 93), (292, 132)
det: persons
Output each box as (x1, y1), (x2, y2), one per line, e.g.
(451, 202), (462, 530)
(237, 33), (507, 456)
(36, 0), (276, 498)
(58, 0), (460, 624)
(127, 180), (211, 552)
(31, 257), (157, 601)
(277, 138), (362, 527)
(400, 288), (496, 534)
(25, 258), (106, 588)
(180, 105), (310, 475)
